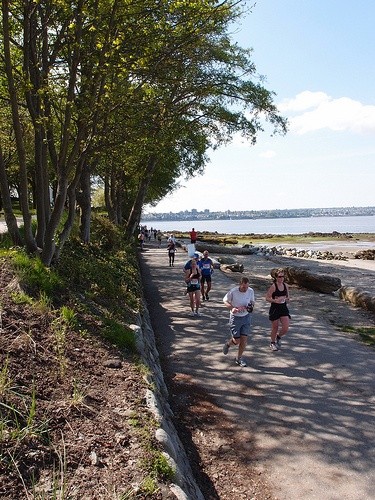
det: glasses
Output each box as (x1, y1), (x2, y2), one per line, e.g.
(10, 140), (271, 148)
(277, 276), (284, 278)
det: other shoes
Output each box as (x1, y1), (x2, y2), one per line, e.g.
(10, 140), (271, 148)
(191, 311), (194, 316)
(203, 296), (205, 300)
(172, 264), (174, 267)
(196, 310), (201, 316)
(170, 264), (171, 267)
(184, 291), (186, 296)
(205, 292), (209, 300)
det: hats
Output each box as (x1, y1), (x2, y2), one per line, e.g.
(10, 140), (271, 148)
(193, 253), (199, 258)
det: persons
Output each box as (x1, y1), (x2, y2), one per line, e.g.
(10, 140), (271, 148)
(139, 225), (214, 316)
(223, 277), (255, 367)
(265, 272), (289, 350)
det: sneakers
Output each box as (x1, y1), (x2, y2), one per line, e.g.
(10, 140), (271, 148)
(270, 343), (278, 351)
(276, 335), (281, 349)
(222, 343), (229, 354)
(236, 356), (246, 366)
